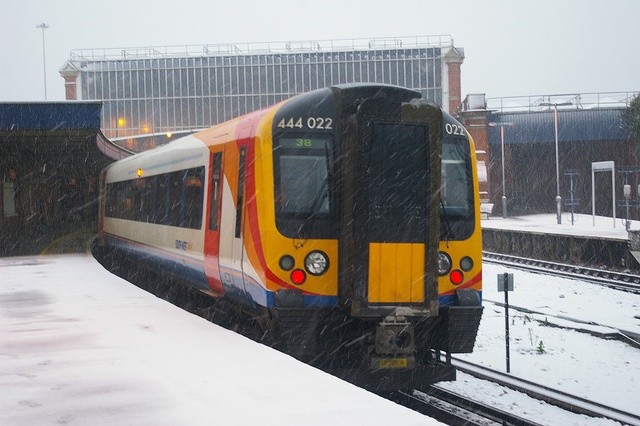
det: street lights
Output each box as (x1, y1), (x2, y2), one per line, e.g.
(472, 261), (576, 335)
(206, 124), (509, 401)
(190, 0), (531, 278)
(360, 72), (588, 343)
(489, 123), (513, 218)
(624, 184), (630, 231)
(539, 102), (572, 224)
(36, 23), (49, 99)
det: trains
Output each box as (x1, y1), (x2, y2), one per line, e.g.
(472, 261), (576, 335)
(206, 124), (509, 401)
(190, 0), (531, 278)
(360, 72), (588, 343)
(100, 82), (484, 391)
